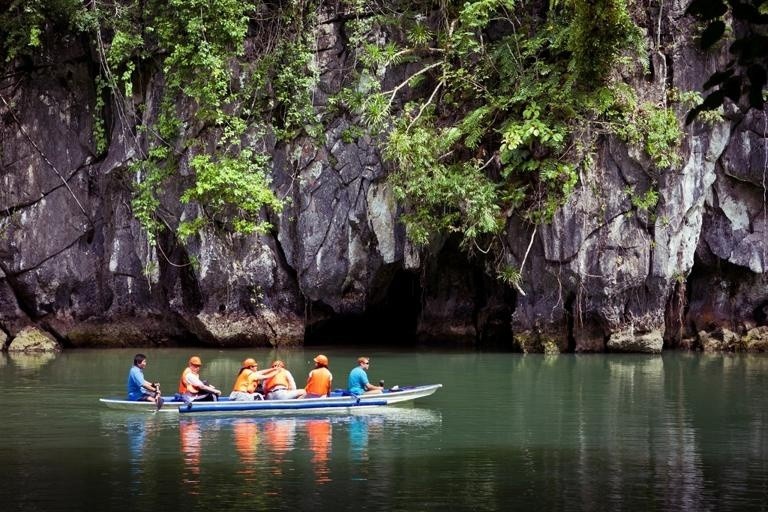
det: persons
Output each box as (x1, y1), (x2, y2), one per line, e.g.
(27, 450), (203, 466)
(262, 359), (307, 399)
(178, 355), (222, 402)
(127, 354), (161, 402)
(229, 358), (281, 400)
(348, 357), (384, 396)
(305, 354), (333, 399)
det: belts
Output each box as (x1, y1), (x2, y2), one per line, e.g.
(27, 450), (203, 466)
(268, 387), (288, 393)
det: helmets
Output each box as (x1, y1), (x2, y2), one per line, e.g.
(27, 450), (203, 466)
(189, 356), (202, 366)
(313, 354), (329, 366)
(272, 360), (285, 368)
(243, 358), (257, 368)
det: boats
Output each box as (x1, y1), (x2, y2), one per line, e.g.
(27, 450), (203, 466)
(100, 384), (442, 411)
(105, 406), (441, 429)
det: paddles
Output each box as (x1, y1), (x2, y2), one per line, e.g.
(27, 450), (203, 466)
(156, 384), (162, 410)
